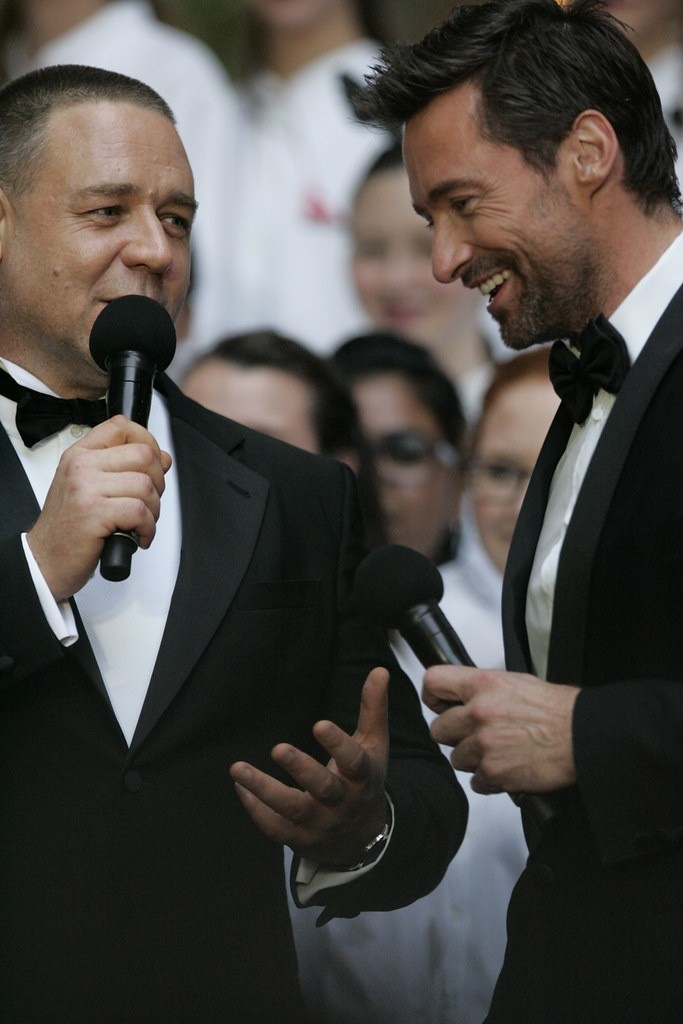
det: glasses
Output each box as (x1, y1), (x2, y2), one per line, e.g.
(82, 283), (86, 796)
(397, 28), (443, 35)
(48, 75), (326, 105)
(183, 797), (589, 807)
(373, 434), (456, 463)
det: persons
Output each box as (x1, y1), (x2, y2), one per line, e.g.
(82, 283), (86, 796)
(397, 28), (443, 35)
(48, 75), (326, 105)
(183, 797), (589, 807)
(373, 0), (682, 1021)
(0, 2), (681, 1023)
(0, 65), (470, 1023)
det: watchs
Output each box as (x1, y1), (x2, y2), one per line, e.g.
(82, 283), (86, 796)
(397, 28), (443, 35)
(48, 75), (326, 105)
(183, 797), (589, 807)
(355, 825), (397, 869)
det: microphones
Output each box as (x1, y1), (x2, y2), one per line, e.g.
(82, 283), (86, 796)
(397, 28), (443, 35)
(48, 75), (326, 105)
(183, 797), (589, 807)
(88, 294), (176, 580)
(352, 543), (564, 828)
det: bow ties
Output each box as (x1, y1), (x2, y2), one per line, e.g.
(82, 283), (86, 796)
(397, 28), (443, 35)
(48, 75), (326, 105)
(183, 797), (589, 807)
(0, 367), (107, 450)
(547, 313), (632, 424)
